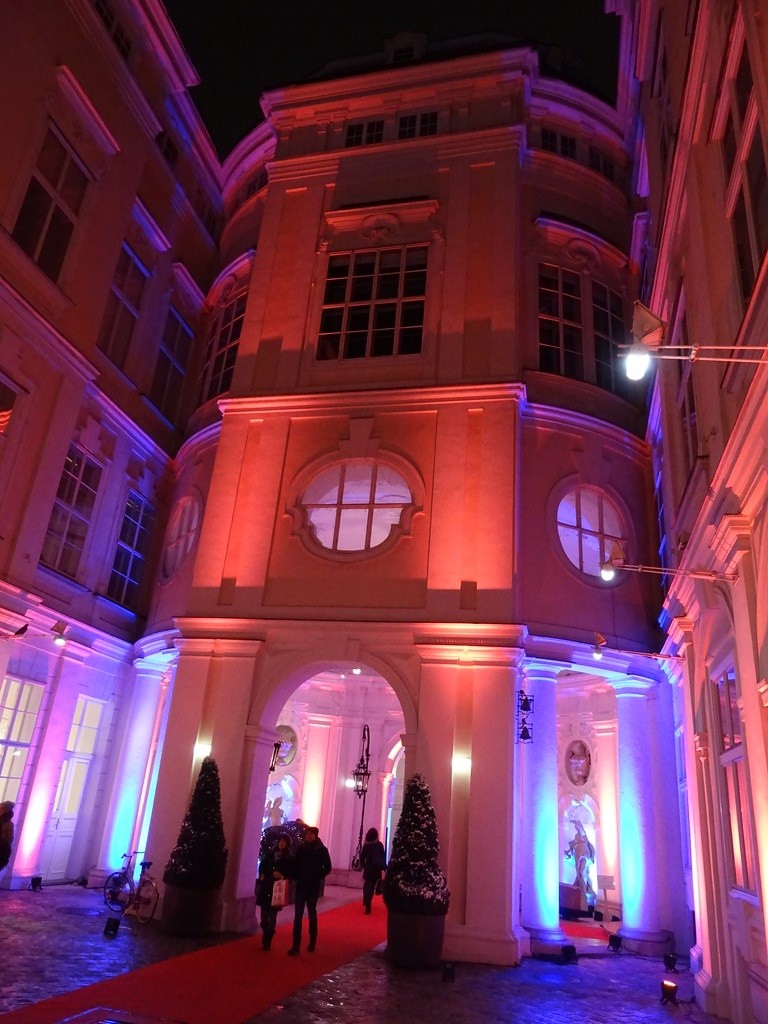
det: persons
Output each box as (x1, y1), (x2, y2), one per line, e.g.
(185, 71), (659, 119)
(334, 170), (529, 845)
(258, 834), (297, 949)
(569, 819), (590, 889)
(264, 797), (283, 825)
(359, 828), (384, 914)
(0, 801), (15, 870)
(292, 827), (333, 954)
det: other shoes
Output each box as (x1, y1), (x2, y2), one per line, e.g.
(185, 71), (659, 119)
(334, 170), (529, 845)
(288, 947), (300, 955)
(262, 927), (276, 950)
(308, 943), (315, 951)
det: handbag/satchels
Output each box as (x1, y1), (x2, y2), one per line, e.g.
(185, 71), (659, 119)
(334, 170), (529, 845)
(255, 878), (260, 896)
(374, 879), (384, 894)
(271, 875), (296, 908)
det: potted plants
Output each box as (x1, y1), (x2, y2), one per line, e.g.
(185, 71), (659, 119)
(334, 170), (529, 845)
(379, 772), (450, 970)
(161, 757), (229, 936)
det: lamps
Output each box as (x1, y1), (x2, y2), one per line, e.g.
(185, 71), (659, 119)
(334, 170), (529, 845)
(561, 945), (579, 964)
(515, 689), (534, 745)
(607, 935), (622, 952)
(663, 953), (679, 974)
(0, 633), (66, 646)
(661, 978), (678, 1007)
(28, 877), (42, 892)
(600, 560), (740, 586)
(104, 917), (120, 936)
(352, 740), (371, 799)
(616, 344), (768, 382)
(593, 631), (608, 660)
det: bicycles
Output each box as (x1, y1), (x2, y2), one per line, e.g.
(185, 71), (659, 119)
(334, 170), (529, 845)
(104, 851), (160, 925)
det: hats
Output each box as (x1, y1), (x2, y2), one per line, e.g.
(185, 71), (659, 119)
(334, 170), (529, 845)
(304, 827), (318, 835)
(277, 834), (292, 845)
